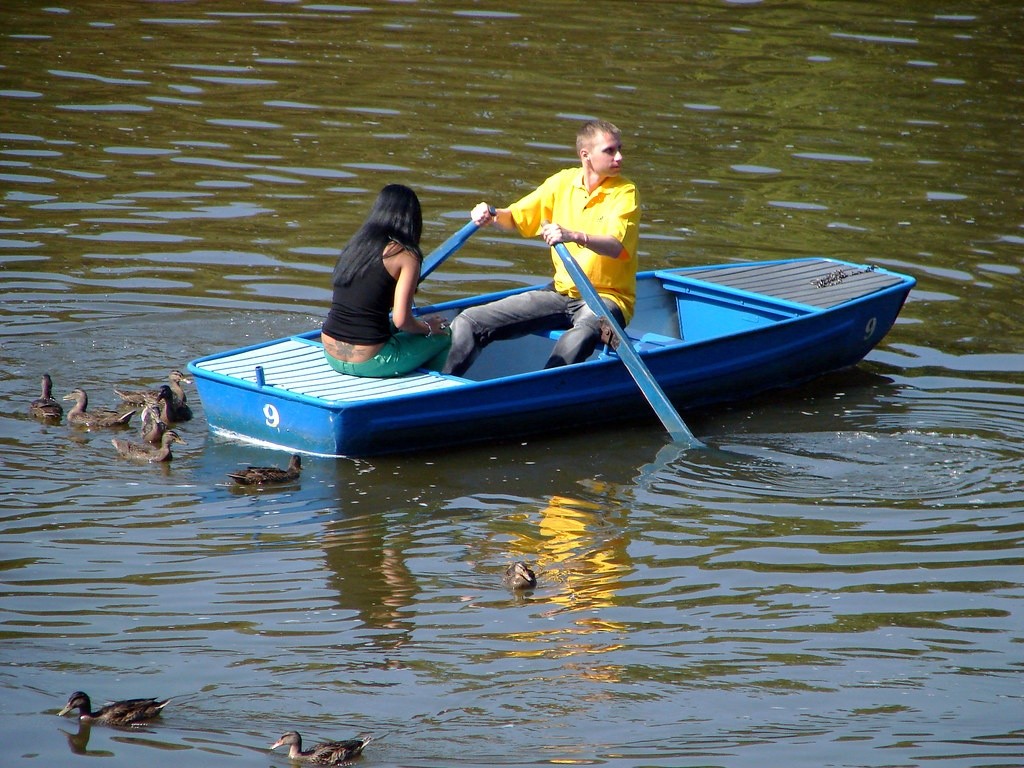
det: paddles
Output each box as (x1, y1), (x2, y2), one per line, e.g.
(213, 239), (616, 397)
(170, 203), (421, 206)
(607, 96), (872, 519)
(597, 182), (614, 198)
(541, 226), (705, 450)
(415, 204), (501, 286)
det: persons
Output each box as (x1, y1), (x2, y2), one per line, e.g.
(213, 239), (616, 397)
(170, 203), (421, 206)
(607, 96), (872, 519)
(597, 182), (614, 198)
(321, 184), (452, 374)
(318, 461), (645, 691)
(443, 120), (641, 377)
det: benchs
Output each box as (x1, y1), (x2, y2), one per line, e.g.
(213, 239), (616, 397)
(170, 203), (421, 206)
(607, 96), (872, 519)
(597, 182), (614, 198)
(531, 327), (685, 357)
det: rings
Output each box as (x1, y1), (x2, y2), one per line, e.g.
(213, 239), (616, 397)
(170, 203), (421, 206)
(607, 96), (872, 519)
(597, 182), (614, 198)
(441, 323), (445, 329)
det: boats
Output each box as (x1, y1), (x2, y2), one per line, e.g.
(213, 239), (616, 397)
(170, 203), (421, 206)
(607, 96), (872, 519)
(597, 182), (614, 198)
(185, 256), (915, 459)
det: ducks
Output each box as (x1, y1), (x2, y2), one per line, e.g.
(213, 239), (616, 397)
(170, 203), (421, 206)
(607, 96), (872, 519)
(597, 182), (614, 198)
(270, 731), (371, 766)
(56, 691), (173, 723)
(31, 370), (193, 462)
(504, 561), (537, 590)
(226, 455), (302, 484)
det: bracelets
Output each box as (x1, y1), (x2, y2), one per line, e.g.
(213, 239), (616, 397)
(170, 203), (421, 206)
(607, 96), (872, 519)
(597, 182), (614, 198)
(423, 320), (433, 338)
(577, 232), (589, 249)
